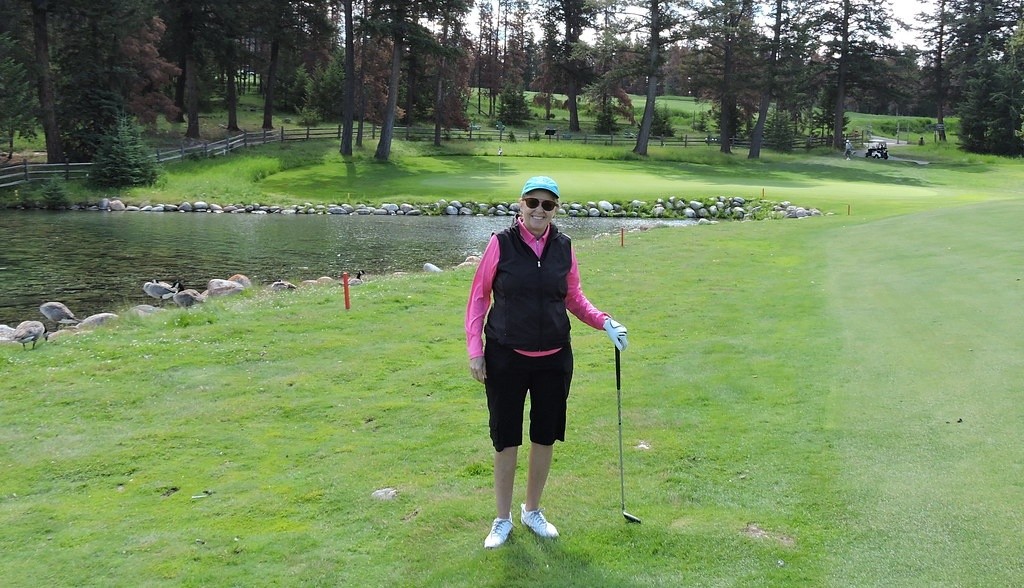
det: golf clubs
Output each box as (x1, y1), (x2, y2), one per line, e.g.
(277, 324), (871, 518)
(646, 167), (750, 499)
(614, 338), (641, 524)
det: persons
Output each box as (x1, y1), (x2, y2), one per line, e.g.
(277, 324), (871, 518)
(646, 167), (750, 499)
(466, 176), (628, 548)
(845, 139), (852, 161)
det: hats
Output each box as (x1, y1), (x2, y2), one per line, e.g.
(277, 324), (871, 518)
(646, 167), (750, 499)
(522, 176), (560, 198)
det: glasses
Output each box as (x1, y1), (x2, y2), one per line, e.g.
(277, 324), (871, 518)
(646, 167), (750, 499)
(521, 197), (559, 211)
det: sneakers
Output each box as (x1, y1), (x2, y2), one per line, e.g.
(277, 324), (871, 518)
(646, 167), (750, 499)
(484, 511), (518, 549)
(520, 503), (559, 538)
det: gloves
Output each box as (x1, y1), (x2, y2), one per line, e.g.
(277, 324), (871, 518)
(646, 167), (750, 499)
(602, 319), (627, 352)
